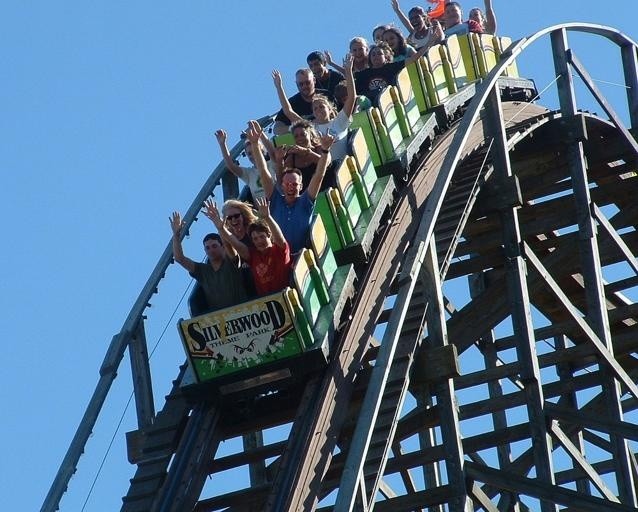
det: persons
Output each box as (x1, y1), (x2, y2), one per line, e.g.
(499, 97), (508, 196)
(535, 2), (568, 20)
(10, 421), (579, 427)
(323, 0), (497, 111)
(167, 50), (356, 310)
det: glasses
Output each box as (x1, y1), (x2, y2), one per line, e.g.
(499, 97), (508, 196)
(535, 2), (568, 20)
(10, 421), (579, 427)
(299, 81), (310, 86)
(227, 213), (242, 220)
(283, 183), (301, 187)
(245, 151), (264, 155)
